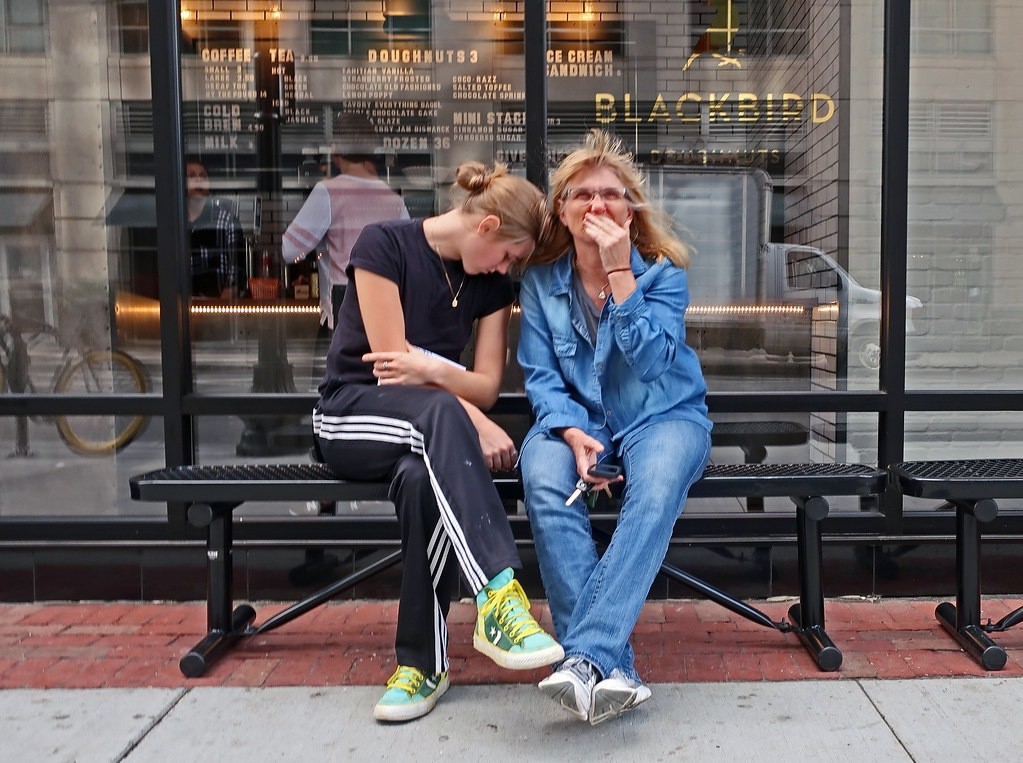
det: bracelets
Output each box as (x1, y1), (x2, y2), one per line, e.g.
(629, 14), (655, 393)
(606, 267), (634, 275)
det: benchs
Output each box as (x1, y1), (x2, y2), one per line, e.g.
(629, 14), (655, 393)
(888, 458), (1023, 671)
(130, 463), (889, 677)
(266, 421), (808, 516)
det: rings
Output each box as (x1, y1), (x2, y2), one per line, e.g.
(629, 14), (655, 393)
(382, 360), (388, 370)
(511, 449), (519, 457)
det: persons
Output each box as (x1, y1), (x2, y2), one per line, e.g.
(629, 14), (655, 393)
(184, 160), (245, 300)
(514, 128), (715, 731)
(309, 155), (565, 723)
(279, 111), (412, 390)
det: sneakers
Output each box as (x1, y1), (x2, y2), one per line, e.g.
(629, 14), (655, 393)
(473, 567), (564, 670)
(538, 657), (600, 721)
(589, 668), (652, 725)
(373, 664), (450, 721)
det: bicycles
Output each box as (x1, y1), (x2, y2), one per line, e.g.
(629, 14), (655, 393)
(1, 276), (152, 457)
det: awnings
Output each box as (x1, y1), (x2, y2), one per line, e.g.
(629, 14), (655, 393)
(907, 185), (1009, 225)
(1, 189), (52, 228)
(91, 188), (255, 229)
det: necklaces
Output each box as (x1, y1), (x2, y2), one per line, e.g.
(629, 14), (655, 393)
(434, 246), (469, 309)
(591, 281), (609, 300)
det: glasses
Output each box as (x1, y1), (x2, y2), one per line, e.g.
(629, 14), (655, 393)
(563, 187), (627, 207)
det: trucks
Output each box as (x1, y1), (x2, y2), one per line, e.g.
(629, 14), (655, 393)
(637, 165), (925, 375)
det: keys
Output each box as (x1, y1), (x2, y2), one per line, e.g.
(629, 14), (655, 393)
(566, 476), (595, 508)
